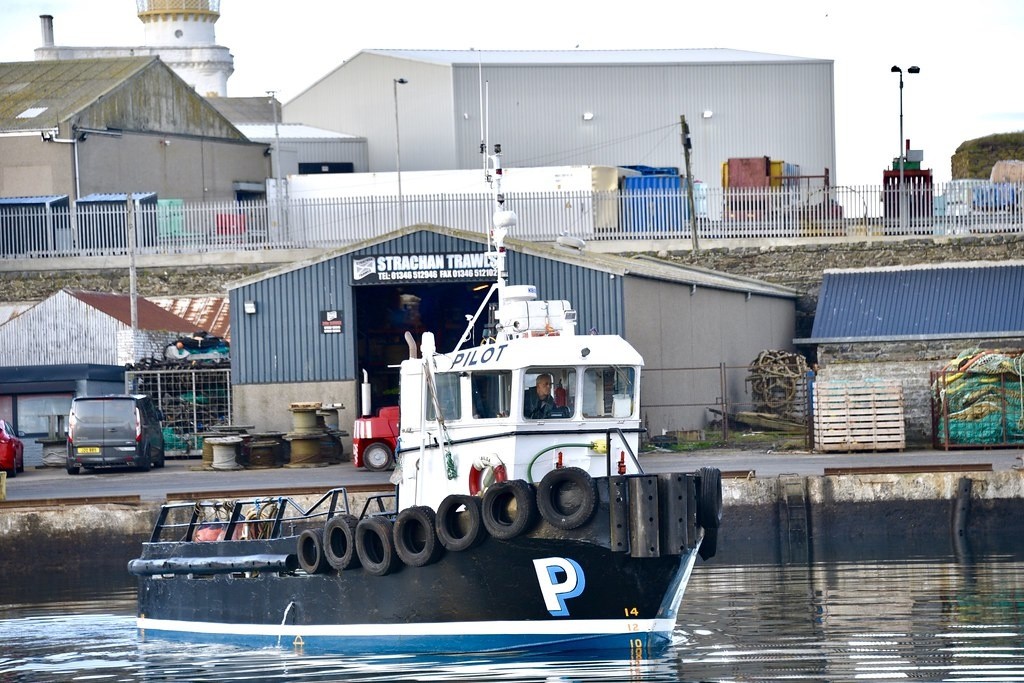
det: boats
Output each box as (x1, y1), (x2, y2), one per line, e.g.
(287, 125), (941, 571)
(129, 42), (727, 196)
(128, 54), (720, 657)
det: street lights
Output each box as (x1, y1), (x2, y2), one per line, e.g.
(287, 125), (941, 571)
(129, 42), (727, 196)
(392, 78), (408, 229)
(891, 65), (921, 182)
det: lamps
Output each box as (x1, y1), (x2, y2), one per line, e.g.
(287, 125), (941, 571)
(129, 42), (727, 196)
(703, 111), (713, 118)
(244, 300), (256, 314)
(584, 111), (594, 121)
(159, 138), (171, 147)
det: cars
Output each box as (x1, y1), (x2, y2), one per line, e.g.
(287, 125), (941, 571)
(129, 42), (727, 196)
(0, 420), (26, 478)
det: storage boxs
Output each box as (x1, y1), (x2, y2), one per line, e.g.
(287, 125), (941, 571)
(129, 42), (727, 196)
(622, 175), (696, 232)
(721, 161), (802, 198)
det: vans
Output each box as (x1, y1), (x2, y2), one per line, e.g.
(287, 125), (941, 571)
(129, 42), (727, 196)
(66, 394), (165, 471)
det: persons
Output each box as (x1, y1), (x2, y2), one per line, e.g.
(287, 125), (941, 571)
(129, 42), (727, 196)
(525, 374), (556, 419)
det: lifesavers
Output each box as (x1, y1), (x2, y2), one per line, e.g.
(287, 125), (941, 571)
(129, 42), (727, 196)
(536, 467), (598, 530)
(435, 494), (486, 551)
(468, 453), (506, 496)
(698, 466), (723, 561)
(392, 506), (439, 567)
(355, 516), (397, 576)
(296, 528), (325, 574)
(322, 513), (359, 570)
(481, 478), (537, 540)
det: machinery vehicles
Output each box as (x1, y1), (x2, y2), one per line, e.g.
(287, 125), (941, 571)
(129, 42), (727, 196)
(353, 365), (400, 473)
